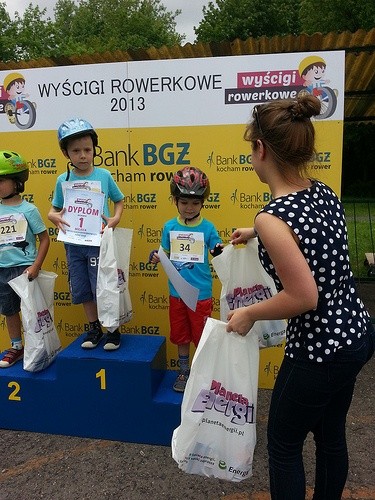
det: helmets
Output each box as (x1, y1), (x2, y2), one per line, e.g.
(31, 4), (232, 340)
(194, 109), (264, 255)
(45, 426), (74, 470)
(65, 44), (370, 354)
(0, 150), (29, 175)
(173, 167), (210, 199)
(58, 118), (94, 144)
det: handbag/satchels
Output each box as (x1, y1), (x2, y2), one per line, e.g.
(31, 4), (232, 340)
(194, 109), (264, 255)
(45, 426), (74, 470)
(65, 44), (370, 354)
(8, 271), (60, 372)
(97, 227), (133, 334)
(211, 236), (286, 349)
(171, 318), (258, 483)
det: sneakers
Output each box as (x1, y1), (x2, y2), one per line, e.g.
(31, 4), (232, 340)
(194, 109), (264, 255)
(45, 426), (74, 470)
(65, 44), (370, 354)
(173, 369), (190, 391)
(104, 328), (120, 350)
(81, 330), (103, 348)
(0, 345), (24, 367)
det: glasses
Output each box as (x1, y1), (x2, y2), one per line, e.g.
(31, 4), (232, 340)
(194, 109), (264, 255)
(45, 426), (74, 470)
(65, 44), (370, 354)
(252, 105), (265, 138)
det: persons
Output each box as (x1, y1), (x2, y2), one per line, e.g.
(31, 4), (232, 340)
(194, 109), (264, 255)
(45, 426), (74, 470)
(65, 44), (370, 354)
(149, 167), (225, 391)
(0, 149), (50, 367)
(227, 94), (375, 500)
(48, 118), (125, 349)
